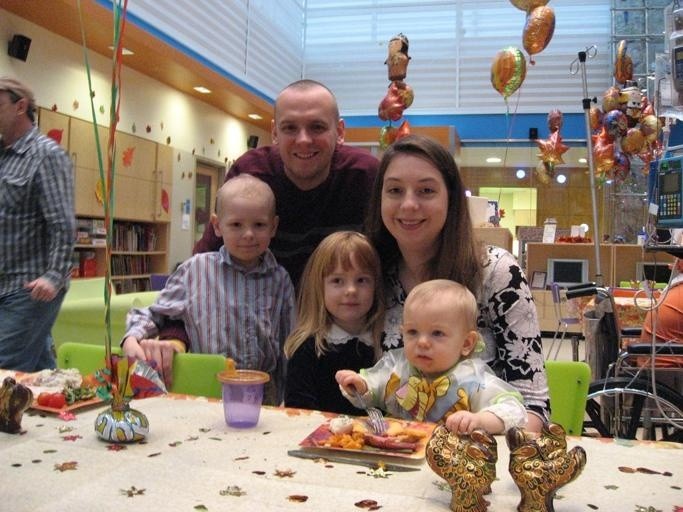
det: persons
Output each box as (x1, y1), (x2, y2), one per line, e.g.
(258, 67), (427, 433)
(635, 272), (682, 444)
(120, 172), (295, 405)
(0, 77), (75, 372)
(669, 228), (682, 276)
(335, 278), (528, 437)
(140, 80), (381, 395)
(283, 230), (384, 417)
(368, 136), (551, 439)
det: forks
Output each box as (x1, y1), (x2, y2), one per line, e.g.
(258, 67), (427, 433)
(346, 383), (386, 435)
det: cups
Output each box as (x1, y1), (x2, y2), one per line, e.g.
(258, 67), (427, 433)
(215, 370), (269, 428)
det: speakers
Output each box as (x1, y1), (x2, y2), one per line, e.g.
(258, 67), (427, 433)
(248, 136), (260, 147)
(9, 34), (32, 61)
(529, 127), (538, 139)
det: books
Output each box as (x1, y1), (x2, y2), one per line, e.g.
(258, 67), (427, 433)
(111, 220), (157, 296)
(72, 251), (97, 278)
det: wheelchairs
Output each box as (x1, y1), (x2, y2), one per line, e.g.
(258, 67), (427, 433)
(565, 282), (682, 444)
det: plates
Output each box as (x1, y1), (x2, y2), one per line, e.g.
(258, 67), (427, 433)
(29, 390), (104, 415)
(298, 416), (436, 460)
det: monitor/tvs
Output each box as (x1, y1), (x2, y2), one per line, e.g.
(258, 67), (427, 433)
(636, 262), (671, 284)
(545, 258), (588, 287)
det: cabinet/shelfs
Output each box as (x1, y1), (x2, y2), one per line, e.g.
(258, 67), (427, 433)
(526, 241), (615, 285)
(37, 218), (169, 293)
(610, 244), (683, 288)
(33, 106), (109, 218)
(109, 127), (173, 219)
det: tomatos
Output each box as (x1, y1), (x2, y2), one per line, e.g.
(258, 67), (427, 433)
(50, 393), (65, 409)
(37, 392), (49, 407)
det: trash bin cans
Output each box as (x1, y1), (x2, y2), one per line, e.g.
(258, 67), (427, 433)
(583, 311), (617, 383)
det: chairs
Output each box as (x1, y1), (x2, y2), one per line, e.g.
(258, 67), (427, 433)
(548, 282), (589, 360)
(539, 359), (591, 438)
(51, 340), (232, 403)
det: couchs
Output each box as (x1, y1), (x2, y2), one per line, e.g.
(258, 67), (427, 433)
(48, 274), (166, 346)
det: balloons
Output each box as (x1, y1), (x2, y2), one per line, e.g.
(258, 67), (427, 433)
(383, 30), (412, 87)
(584, 38), (663, 191)
(521, 7), (556, 64)
(376, 81), (415, 122)
(535, 110), (569, 185)
(377, 120), (410, 151)
(510, 2), (550, 15)
(489, 44), (526, 102)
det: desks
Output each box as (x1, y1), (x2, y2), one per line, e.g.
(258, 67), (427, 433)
(524, 284), (664, 336)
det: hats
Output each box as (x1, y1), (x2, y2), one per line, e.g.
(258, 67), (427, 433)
(1, 77), (33, 104)
